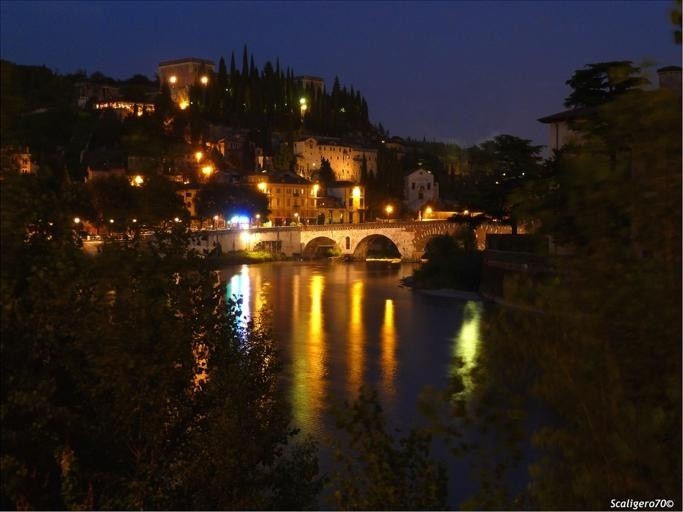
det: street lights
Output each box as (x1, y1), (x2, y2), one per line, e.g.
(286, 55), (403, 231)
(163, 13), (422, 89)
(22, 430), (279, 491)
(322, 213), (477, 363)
(294, 212), (298, 226)
(386, 207), (392, 223)
